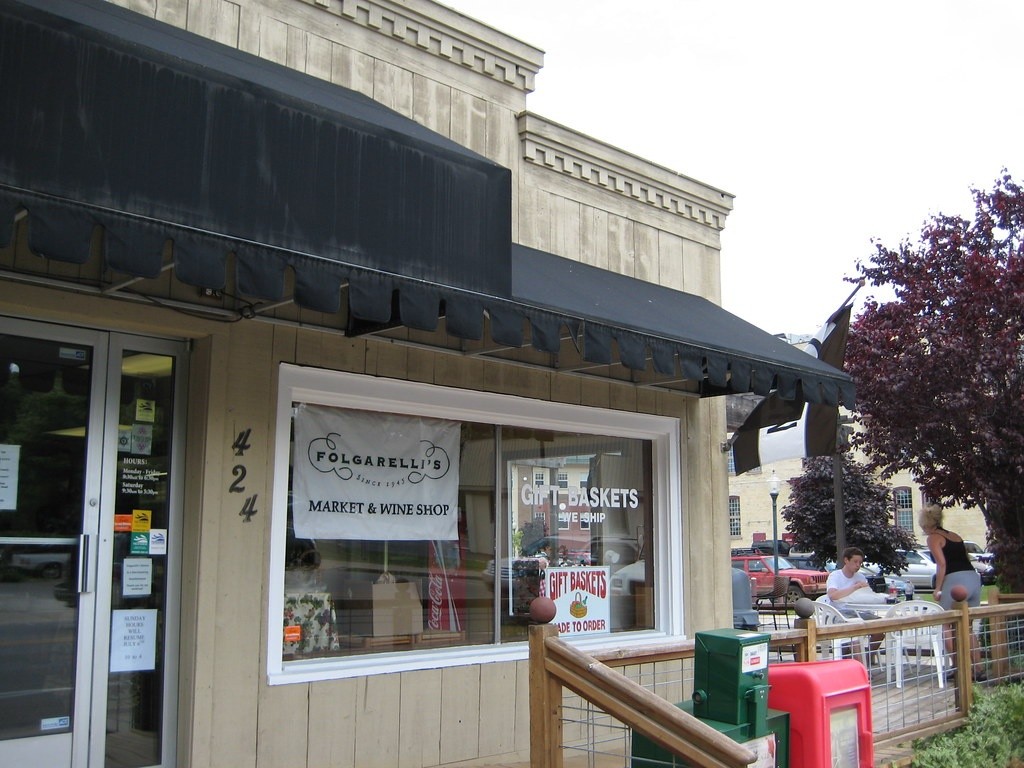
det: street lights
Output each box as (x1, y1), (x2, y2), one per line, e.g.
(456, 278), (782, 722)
(767, 468), (783, 578)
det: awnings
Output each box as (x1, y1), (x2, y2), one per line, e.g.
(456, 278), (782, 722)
(512, 242), (855, 411)
(0, 0), (512, 347)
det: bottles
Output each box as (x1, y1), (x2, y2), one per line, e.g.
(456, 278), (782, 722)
(889, 581), (896, 597)
(905, 580), (913, 593)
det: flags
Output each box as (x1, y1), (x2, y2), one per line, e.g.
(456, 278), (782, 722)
(732, 292), (858, 477)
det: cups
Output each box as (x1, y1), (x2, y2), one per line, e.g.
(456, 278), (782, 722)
(905, 594), (913, 601)
(887, 599), (895, 604)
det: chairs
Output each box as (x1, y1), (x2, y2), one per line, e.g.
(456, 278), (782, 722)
(751, 576), (791, 630)
(811, 593), (946, 688)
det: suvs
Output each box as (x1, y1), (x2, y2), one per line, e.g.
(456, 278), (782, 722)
(782, 556), (887, 592)
(732, 547), (831, 609)
(482, 534), (643, 600)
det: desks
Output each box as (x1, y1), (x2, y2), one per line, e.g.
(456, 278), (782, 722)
(843, 603), (912, 675)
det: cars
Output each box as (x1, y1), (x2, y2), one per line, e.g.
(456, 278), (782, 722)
(607, 556), (645, 627)
(862, 540), (997, 589)
(862, 561), (915, 601)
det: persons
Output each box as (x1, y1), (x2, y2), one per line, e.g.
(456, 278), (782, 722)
(826, 547), (886, 684)
(919, 504), (988, 681)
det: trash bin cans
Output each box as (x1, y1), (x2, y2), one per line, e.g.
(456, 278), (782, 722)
(631, 624), (880, 768)
(731, 567), (759, 631)
